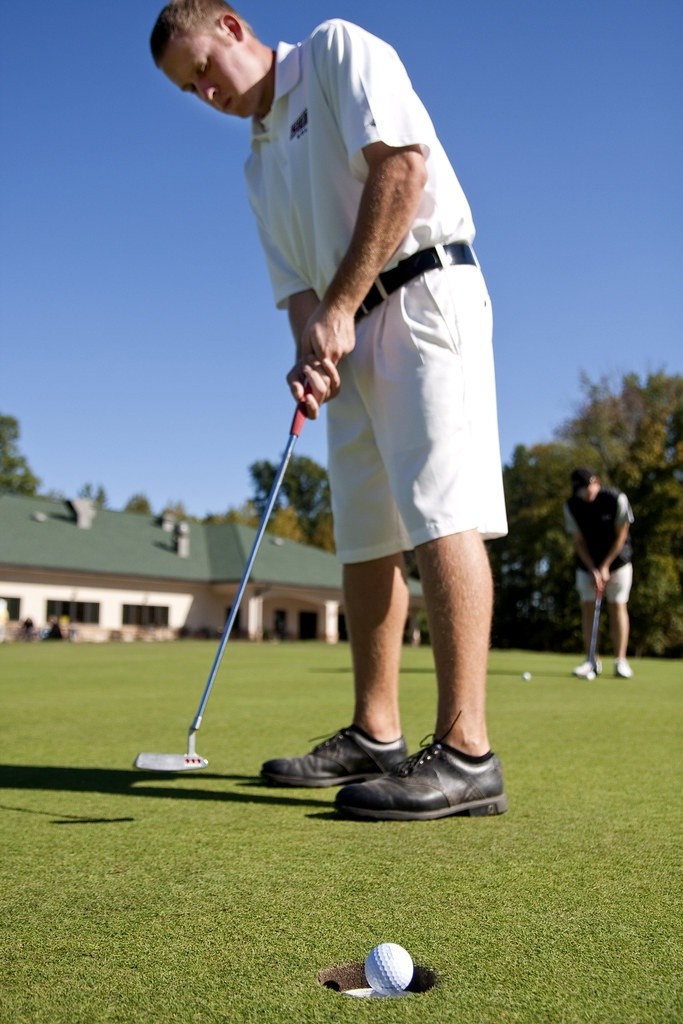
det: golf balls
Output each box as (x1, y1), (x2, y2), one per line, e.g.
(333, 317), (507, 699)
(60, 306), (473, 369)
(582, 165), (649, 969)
(364, 943), (415, 995)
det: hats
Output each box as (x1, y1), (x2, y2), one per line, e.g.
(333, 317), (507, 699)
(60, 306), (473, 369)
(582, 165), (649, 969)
(570, 467), (592, 496)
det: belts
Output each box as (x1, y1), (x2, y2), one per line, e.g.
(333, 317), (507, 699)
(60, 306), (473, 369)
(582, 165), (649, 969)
(354, 243), (478, 324)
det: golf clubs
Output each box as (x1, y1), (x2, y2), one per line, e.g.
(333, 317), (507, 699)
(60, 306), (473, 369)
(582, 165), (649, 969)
(135, 372), (312, 771)
(585, 590), (600, 678)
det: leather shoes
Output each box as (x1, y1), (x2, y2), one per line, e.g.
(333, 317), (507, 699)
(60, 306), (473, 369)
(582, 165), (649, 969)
(260, 727), (409, 789)
(333, 711), (509, 820)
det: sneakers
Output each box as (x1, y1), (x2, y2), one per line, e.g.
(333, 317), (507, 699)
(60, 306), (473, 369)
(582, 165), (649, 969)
(573, 660), (603, 679)
(616, 657), (632, 678)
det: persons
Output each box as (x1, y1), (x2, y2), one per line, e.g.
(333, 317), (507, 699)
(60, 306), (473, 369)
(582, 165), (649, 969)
(562, 467), (635, 680)
(150, 1), (508, 820)
(22, 615), (63, 642)
